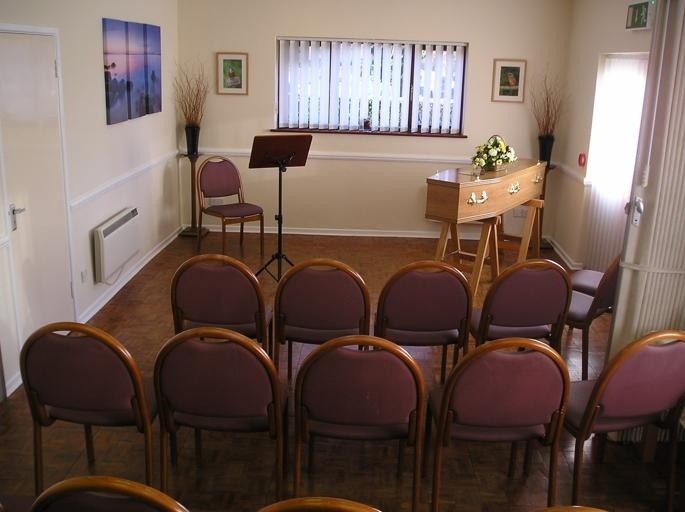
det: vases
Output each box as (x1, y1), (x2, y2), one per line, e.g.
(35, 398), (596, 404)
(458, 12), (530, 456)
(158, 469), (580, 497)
(537, 133), (555, 164)
(182, 122), (200, 152)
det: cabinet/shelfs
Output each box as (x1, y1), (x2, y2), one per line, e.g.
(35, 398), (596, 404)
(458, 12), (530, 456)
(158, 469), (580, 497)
(425, 155), (550, 308)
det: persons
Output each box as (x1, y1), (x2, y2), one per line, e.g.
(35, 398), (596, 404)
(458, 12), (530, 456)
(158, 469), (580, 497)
(507, 71), (515, 87)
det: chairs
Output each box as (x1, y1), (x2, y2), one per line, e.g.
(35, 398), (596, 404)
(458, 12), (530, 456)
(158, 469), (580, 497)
(194, 154), (267, 262)
(19, 252), (684, 512)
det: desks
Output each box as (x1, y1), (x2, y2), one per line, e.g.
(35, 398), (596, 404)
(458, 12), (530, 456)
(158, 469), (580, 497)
(529, 161), (556, 252)
(179, 151), (210, 240)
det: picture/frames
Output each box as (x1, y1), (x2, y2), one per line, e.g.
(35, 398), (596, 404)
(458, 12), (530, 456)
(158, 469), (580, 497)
(491, 56), (528, 106)
(213, 49), (251, 99)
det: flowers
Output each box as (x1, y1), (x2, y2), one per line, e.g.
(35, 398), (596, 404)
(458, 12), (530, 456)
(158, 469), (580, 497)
(475, 137), (518, 173)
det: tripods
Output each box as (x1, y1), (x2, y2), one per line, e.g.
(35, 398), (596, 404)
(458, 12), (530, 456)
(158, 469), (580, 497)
(255, 166), (295, 283)
